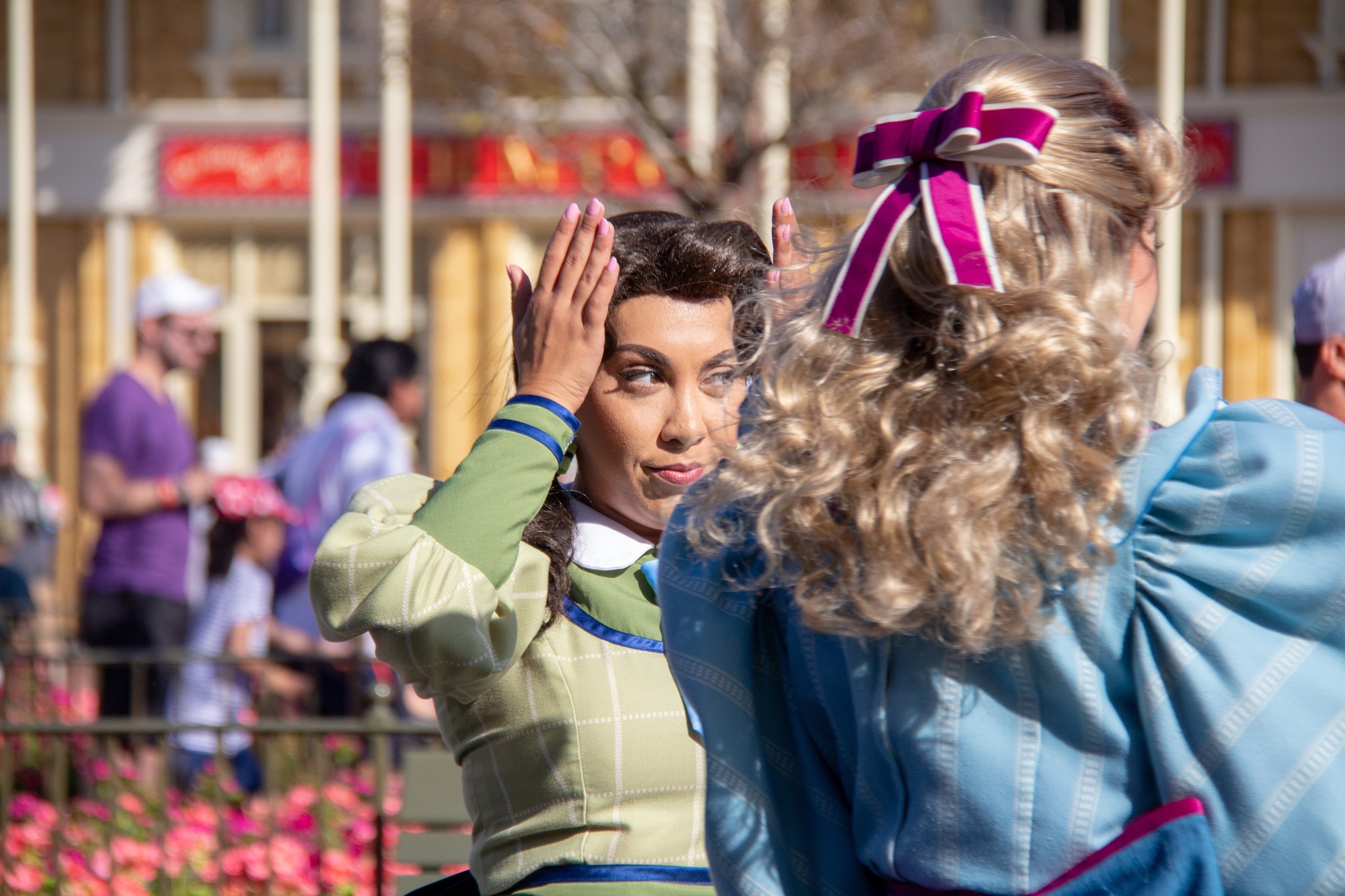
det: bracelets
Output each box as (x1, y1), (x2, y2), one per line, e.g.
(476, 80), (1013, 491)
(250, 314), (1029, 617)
(155, 472), (181, 509)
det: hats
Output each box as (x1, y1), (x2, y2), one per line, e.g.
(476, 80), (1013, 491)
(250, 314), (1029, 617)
(1291, 250), (1345, 344)
(136, 269), (222, 325)
(208, 476), (305, 527)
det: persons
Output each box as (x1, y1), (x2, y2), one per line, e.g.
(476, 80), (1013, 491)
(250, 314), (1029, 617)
(280, 197), (811, 896)
(169, 476), (353, 797)
(1289, 251), (1345, 422)
(276, 338), (432, 723)
(0, 425), (55, 640)
(79, 271), (263, 752)
(654, 44), (1342, 896)
